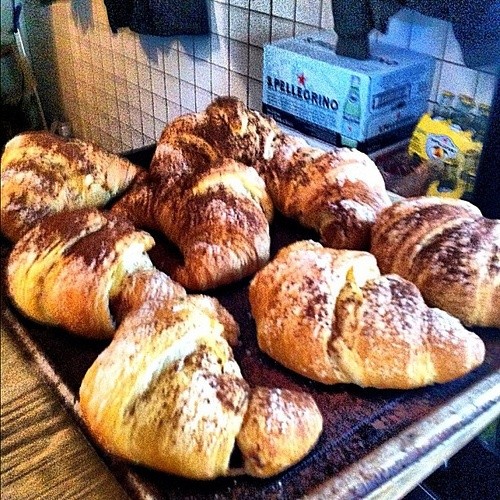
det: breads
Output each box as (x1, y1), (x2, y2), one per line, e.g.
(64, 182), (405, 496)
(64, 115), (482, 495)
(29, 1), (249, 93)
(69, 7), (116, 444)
(250, 239), (485, 390)
(78, 294), (324, 480)
(0, 129), (145, 246)
(109, 97), (392, 291)
(368, 195), (500, 329)
(6, 206), (186, 337)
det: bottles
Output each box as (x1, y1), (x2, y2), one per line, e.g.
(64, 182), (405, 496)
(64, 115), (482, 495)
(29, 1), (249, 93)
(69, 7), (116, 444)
(341, 75), (361, 139)
(412, 90), (491, 196)
